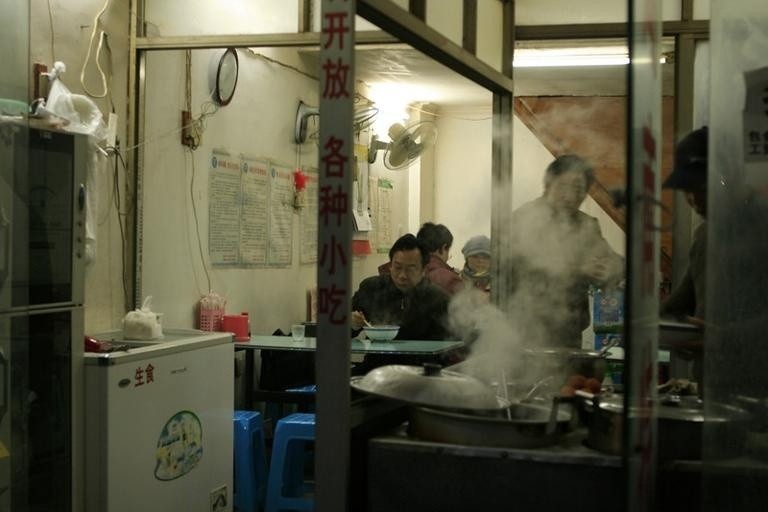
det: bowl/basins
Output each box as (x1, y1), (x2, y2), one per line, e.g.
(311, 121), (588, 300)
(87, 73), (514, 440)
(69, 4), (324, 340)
(362, 324), (400, 343)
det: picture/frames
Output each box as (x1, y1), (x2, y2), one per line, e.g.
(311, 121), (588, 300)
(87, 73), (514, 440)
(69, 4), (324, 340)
(209, 47), (240, 108)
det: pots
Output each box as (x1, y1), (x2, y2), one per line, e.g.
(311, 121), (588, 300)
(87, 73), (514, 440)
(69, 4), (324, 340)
(406, 390), (587, 448)
(568, 388), (761, 456)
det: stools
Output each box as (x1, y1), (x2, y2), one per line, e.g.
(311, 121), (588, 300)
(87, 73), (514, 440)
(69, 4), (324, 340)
(266, 413), (316, 511)
(234, 410), (265, 511)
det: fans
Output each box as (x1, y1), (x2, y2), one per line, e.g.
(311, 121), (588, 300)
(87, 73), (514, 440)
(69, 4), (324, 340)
(292, 92), (379, 147)
(365, 119), (441, 172)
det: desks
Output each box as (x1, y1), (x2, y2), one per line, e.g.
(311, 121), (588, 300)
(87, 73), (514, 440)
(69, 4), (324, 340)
(235, 336), (467, 415)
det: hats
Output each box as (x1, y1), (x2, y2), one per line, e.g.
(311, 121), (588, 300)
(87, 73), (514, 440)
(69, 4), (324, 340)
(463, 237), (491, 259)
(662, 125), (706, 188)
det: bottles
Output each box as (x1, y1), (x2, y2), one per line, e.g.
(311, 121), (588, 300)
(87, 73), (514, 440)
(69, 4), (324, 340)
(600, 369), (613, 399)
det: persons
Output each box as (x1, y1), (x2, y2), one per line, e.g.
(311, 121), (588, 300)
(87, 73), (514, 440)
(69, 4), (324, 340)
(459, 234), (491, 300)
(352, 233), (455, 372)
(377, 220), (467, 307)
(658, 127), (768, 395)
(510, 155), (626, 349)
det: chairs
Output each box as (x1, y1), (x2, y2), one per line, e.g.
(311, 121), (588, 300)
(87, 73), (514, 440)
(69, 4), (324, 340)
(285, 323), (317, 414)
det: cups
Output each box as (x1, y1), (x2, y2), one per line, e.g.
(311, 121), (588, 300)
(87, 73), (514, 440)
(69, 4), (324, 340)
(292, 324), (306, 343)
(221, 315), (250, 342)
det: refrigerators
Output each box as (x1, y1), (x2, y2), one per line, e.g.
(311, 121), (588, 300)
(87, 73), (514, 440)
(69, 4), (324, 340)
(71, 325), (234, 510)
